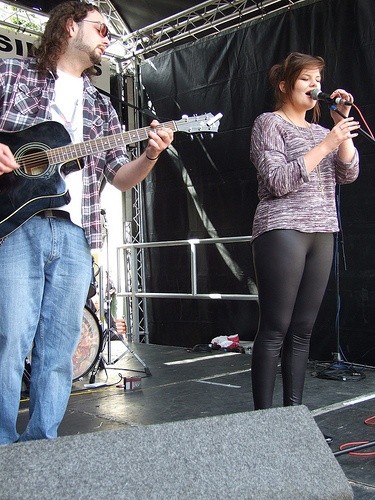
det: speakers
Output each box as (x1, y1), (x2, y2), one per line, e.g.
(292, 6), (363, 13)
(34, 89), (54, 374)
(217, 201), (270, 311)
(0, 404), (355, 500)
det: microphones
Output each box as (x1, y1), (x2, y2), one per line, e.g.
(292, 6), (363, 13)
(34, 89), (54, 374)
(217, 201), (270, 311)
(310, 89), (352, 105)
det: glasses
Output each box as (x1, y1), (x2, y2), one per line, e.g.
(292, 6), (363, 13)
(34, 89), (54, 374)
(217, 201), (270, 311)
(78, 20), (112, 41)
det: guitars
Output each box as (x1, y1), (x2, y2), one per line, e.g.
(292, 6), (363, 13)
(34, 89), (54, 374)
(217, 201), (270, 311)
(0, 112), (224, 238)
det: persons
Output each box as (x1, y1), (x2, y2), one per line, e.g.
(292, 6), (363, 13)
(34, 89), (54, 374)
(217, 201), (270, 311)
(0, 2), (174, 447)
(247, 52), (359, 443)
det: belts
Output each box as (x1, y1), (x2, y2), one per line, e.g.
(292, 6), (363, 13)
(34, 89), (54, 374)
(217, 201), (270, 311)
(35, 209), (70, 220)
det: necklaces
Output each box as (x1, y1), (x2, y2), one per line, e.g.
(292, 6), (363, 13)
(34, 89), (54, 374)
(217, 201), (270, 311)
(281, 109), (325, 196)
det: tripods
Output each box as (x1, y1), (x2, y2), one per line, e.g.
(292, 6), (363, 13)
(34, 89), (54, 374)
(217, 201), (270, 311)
(90, 209), (152, 383)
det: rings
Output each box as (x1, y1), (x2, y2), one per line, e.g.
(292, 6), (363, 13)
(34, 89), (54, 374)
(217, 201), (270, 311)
(339, 126), (344, 130)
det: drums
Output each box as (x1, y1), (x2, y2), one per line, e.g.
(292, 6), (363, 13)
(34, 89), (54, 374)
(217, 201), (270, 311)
(23, 305), (104, 384)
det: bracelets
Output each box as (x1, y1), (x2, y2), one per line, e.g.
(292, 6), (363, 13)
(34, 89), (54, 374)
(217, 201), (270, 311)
(145, 151), (159, 161)
